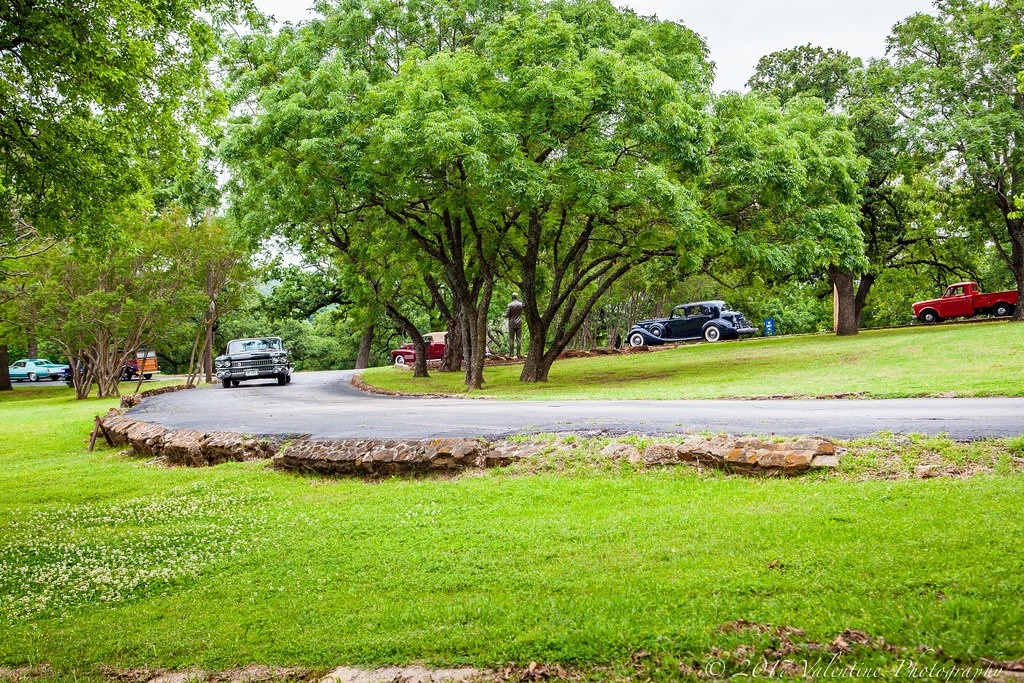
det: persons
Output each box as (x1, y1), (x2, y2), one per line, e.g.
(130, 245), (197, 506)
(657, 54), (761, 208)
(502, 292), (523, 358)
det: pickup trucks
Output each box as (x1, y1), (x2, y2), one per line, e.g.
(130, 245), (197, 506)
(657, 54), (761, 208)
(911, 281), (1019, 323)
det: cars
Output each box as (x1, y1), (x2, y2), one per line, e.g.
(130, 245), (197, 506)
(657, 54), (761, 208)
(8, 358), (70, 382)
(624, 299), (756, 347)
(65, 348), (162, 388)
(214, 336), (291, 388)
(391, 331), (448, 366)
